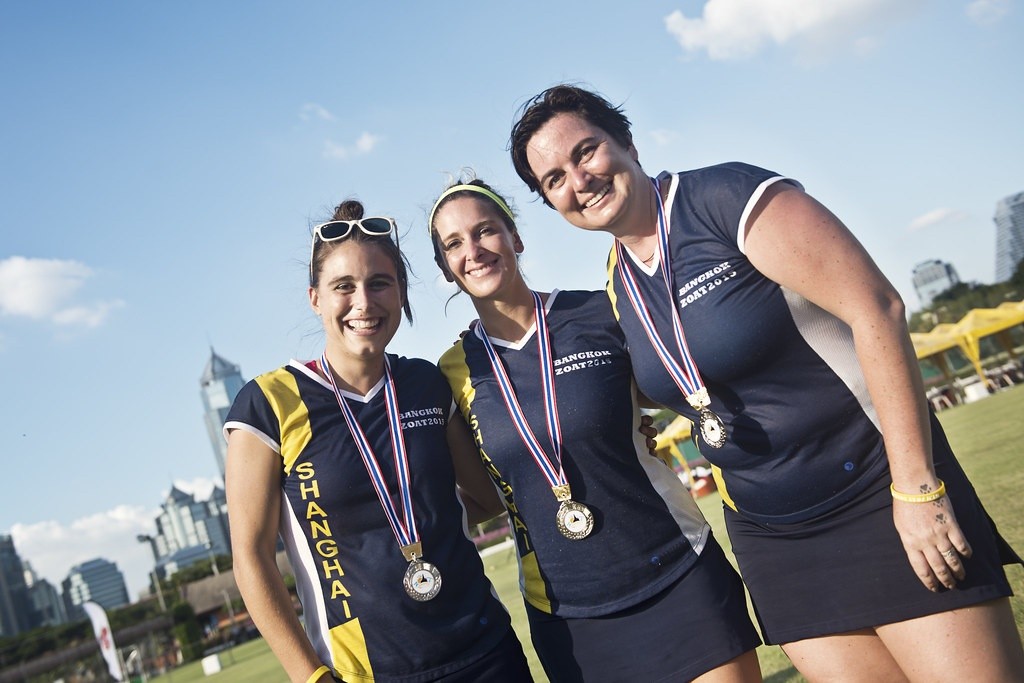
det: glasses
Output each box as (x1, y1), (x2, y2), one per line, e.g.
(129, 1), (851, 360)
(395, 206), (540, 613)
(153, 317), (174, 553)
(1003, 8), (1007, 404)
(310, 216), (400, 283)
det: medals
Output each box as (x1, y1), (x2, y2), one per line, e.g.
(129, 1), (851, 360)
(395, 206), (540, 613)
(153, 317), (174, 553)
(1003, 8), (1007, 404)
(555, 500), (596, 541)
(695, 409), (727, 449)
(401, 561), (443, 600)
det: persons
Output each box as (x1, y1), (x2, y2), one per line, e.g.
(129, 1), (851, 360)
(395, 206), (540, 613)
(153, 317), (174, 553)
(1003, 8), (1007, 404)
(223, 198), (658, 683)
(427, 176), (765, 683)
(505, 84), (1024, 683)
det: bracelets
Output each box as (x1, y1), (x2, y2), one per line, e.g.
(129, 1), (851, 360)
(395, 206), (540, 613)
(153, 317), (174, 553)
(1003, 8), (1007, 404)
(300, 665), (331, 683)
(891, 480), (948, 503)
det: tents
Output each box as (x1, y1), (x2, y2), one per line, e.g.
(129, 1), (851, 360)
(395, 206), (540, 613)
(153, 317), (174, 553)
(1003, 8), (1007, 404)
(652, 412), (695, 496)
(908, 300), (1024, 391)
